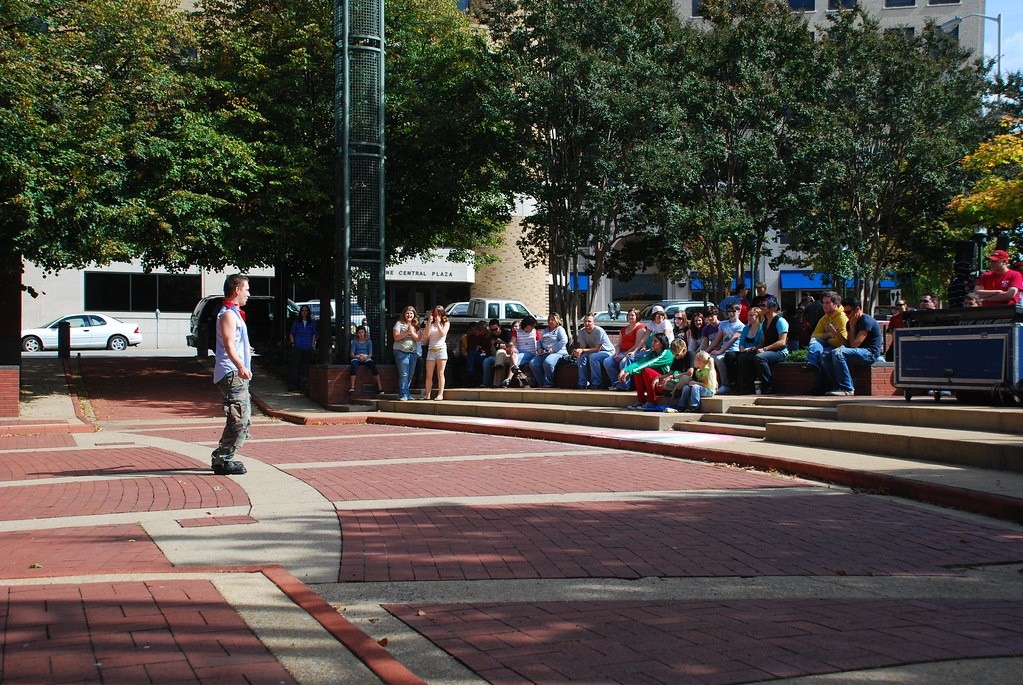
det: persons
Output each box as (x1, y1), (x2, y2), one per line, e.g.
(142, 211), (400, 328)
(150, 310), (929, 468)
(919, 291), (951, 396)
(782, 292), (825, 352)
(964, 292), (982, 306)
(974, 250), (1023, 306)
(212, 273), (253, 475)
(459, 281), (790, 413)
(885, 299), (908, 361)
(805, 292), (849, 395)
(393, 306), (421, 401)
(349, 325), (385, 394)
(821, 296), (882, 395)
(289, 305), (317, 393)
(422, 304), (450, 401)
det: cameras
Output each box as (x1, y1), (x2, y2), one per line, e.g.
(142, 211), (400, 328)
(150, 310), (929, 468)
(431, 313), (437, 317)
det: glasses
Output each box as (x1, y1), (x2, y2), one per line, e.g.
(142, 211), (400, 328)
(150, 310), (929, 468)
(896, 304), (905, 306)
(491, 325), (499, 331)
(728, 310), (736, 313)
(674, 317), (683, 320)
(843, 309), (852, 314)
(796, 311), (802, 314)
(759, 303), (768, 307)
(919, 300), (930, 303)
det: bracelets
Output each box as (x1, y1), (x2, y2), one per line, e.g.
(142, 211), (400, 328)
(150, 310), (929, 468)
(291, 342), (294, 344)
(763, 347), (767, 351)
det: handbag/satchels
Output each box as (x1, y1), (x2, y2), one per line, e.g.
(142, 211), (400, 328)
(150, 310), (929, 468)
(559, 353), (580, 368)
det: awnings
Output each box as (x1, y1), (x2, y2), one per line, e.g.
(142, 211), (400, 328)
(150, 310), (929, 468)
(690, 271), (758, 290)
(570, 271), (589, 290)
(779, 268), (900, 288)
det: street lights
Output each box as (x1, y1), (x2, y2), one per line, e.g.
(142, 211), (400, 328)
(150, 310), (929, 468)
(971, 232), (988, 270)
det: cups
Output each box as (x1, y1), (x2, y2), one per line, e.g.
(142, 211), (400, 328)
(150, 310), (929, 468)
(755, 380), (762, 395)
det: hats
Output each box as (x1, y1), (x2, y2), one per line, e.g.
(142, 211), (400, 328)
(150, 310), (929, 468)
(652, 305), (665, 315)
(987, 249), (1008, 262)
(754, 281), (767, 289)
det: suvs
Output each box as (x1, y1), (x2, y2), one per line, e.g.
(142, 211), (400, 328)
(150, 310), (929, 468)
(185, 295), (336, 351)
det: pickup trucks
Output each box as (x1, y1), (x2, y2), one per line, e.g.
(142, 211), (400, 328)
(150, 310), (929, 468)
(420, 298), (544, 323)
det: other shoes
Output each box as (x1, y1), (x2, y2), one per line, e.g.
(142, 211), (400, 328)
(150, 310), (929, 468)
(718, 386), (731, 394)
(423, 390), (432, 400)
(764, 387), (779, 394)
(608, 385), (622, 390)
(799, 362), (820, 372)
(376, 389), (384, 394)
(407, 393), (418, 399)
(689, 406), (700, 410)
(349, 387), (355, 392)
(399, 395), (408, 401)
(637, 403), (658, 410)
(677, 406), (685, 410)
(488, 364), (503, 369)
(500, 378), (511, 386)
(434, 395), (444, 400)
(511, 364), (520, 373)
(627, 403), (646, 410)
(805, 387), (825, 395)
(824, 388), (855, 395)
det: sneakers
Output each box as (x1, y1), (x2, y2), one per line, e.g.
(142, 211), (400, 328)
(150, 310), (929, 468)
(211, 460), (243, 469)
(214, 461), (246, 474)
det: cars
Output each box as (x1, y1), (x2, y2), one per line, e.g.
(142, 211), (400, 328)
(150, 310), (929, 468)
(639, 300), (714, 328)
(580, 311), (627, 320)
(297, 299), (367, 331)
(21, 313), (141, 351)
(445, 302), (470, 316)
